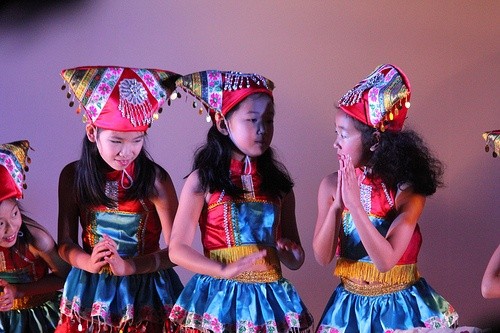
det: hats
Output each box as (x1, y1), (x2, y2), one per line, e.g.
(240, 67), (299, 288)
(482, 130), (500, 158)
(0, 139), (36, 201)
(60, 66), (184, 132)
(176, 70), (275, 123)
(337, 63), (411, 132)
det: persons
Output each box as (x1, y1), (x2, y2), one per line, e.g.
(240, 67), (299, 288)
(54, 65), (185, 333)
(0, 140), (72, 333)
(168, 70), (315, 333)
(313, 64), (459, 333)
(481, 129), (500, 299)
